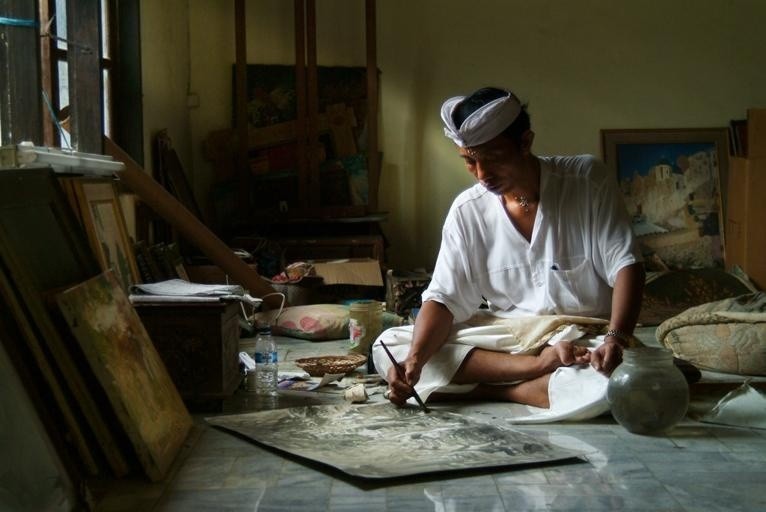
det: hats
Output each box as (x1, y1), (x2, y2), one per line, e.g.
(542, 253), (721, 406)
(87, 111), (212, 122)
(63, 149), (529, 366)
(440, 92), (522, 148)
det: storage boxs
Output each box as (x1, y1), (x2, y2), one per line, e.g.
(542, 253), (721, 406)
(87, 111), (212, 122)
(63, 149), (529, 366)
(721, 104), (763, 287)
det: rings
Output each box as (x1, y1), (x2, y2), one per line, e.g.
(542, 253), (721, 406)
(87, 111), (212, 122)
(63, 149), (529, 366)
(384, 389), (391, 400)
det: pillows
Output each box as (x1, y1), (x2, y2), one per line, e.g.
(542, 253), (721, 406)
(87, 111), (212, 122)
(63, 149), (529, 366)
(247, 304), (407, 341)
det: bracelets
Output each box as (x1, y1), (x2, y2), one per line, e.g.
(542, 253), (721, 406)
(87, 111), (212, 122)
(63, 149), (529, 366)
(602, 327), (629, 344)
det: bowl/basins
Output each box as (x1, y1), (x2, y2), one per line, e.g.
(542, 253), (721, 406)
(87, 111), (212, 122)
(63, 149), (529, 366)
(291, 210), (389, 238)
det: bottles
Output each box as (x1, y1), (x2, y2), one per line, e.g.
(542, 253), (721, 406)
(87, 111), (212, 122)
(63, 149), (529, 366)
(606, 349), (689, 433)
(255, 323), (278, 397)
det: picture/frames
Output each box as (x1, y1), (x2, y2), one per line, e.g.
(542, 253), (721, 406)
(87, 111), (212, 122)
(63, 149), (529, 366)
(1, 167), (137, 470)
(596, 126), (732, 259)
(73, 178), (146, 299)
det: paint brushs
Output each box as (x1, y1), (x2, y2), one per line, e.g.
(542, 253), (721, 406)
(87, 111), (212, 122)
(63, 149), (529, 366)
(380, 339), (430, 413)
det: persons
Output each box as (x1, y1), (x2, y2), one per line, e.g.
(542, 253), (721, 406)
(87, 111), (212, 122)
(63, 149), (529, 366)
(369, 84), (649, 426)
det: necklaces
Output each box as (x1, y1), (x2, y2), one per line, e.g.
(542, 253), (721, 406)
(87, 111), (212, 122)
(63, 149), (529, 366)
(512, 195), (529, 211)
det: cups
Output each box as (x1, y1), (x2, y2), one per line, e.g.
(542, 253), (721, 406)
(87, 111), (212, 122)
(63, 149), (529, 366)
(348, 301), (383, 360)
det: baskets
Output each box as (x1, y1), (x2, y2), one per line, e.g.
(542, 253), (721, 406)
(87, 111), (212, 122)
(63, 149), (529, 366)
(293, 354), (368, 377)
